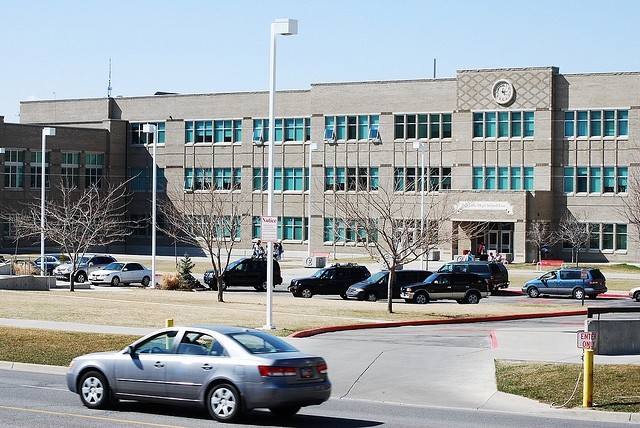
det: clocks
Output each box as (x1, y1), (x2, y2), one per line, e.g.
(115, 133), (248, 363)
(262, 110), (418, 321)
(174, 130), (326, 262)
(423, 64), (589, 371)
(492, 79), (514, 105)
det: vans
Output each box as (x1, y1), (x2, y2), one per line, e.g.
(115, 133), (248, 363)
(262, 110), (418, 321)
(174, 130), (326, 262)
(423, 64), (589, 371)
(345, 266), (433, 302)
(521, 266), (608, 299)
(436, 260), (510, 295)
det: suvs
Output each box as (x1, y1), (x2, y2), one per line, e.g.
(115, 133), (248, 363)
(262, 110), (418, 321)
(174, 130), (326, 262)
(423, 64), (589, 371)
(399, 270), (493, 305)
(202, 256), (283, 292)
(53, 254), (118, 284)
(289, 264), (371, 299)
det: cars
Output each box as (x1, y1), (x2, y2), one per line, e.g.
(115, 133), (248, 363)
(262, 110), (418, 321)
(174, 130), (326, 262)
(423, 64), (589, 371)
(88, 260), (153, 287)
(0, 258), (40, 274)
(33, 255), (70, 273)
(64, 322), (332, 425)
(628, 285), (640, 302)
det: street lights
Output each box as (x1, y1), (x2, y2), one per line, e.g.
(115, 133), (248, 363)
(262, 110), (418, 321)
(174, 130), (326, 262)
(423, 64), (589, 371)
(142, 123), (159, 290)
(412, 141), (426, 271)
(306, 142), (318, 256)
(256, 16), (300, 331)
(40, 126), (56, 277)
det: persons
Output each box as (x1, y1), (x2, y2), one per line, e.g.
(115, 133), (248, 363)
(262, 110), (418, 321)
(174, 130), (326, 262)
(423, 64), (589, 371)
(494, 253), (501, 262)
(251, 240), (268, 260)
(489, 252), (494, 262)
(273, 243), (283, 260)
(467, 252), (472, 261)
